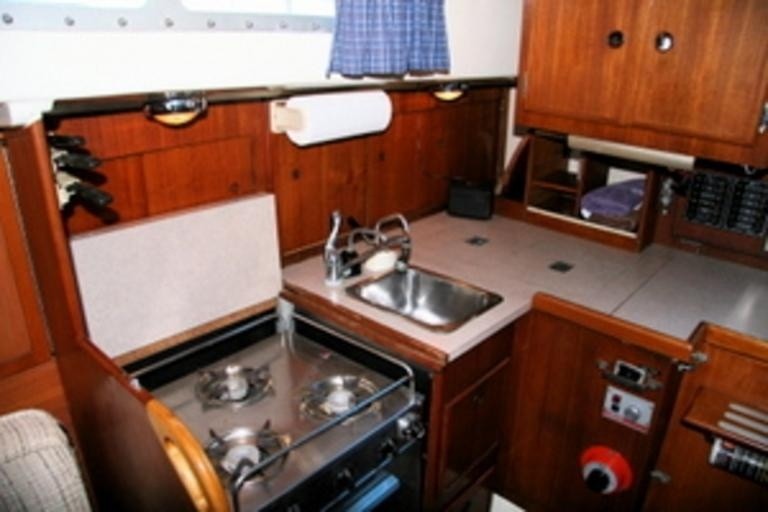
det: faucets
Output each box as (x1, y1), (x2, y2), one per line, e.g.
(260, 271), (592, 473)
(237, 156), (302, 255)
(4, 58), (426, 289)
(339, 234), (412, 278)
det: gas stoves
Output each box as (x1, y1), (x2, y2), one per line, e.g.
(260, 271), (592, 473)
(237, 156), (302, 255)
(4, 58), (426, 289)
(145, 328), (426, 511)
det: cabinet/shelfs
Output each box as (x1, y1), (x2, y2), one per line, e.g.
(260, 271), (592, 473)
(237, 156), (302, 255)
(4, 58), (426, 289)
(513, 1), (764, 171)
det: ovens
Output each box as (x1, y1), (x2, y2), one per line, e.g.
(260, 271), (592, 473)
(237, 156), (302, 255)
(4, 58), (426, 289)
(318, 438), (429, 510)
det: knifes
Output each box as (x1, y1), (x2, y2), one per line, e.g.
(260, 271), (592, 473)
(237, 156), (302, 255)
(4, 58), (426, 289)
(48, 132), (113, 222)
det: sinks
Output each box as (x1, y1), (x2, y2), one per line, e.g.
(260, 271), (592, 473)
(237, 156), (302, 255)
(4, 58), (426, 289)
(345, 263), (505, 334)
